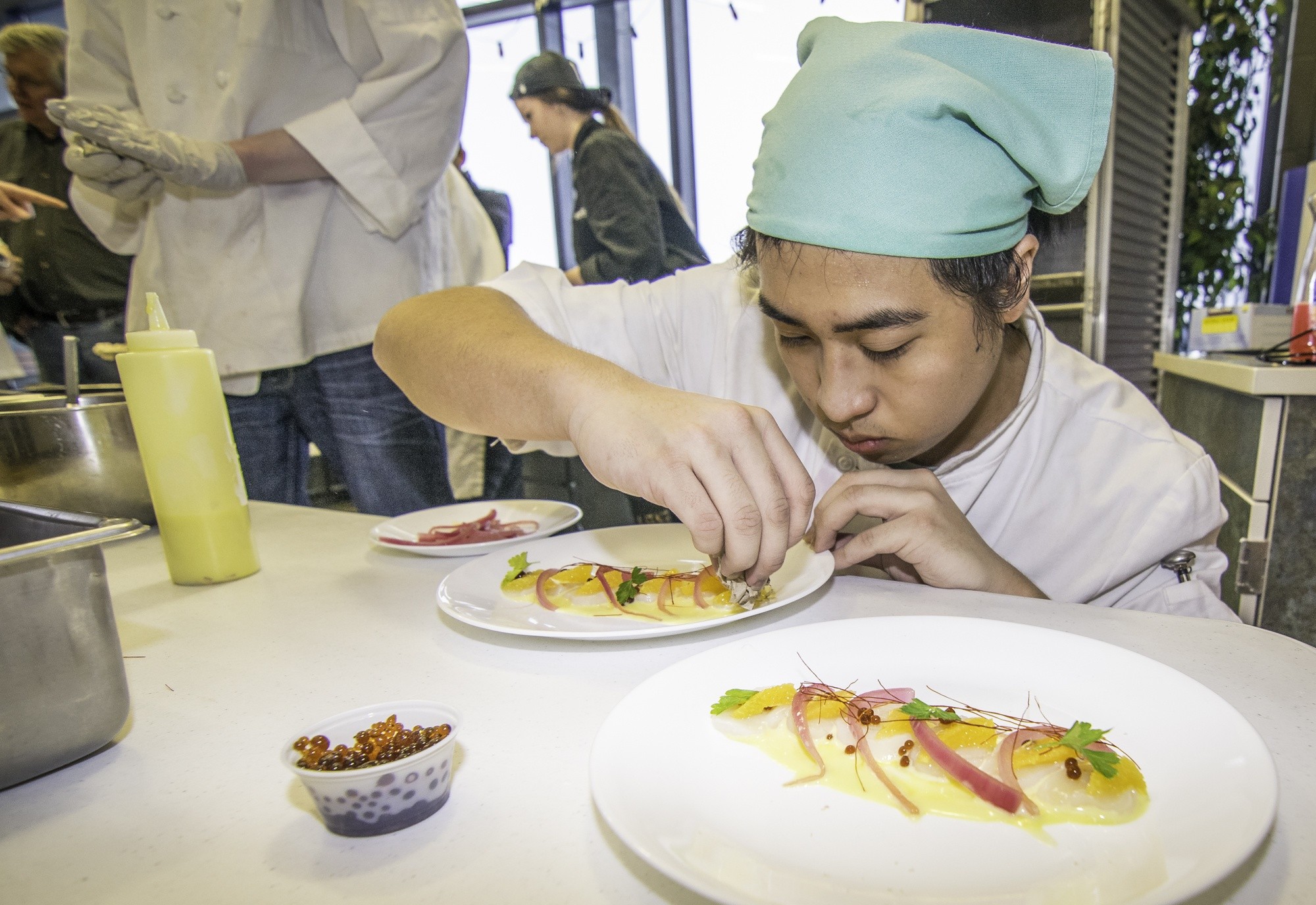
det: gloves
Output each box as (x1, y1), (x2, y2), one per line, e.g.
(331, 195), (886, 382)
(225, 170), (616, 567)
(46, 95), (247, 192)
(62, 145), (165, 203)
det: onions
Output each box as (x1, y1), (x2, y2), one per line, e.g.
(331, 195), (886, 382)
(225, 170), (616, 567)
(786, 683), (1117, 813)
(536, 566), (712, 621)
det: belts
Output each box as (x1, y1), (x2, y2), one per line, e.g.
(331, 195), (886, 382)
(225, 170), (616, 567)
(22, 303), (121, 329)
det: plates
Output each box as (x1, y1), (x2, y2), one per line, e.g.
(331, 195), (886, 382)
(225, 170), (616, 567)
(367, 499), (584, 558)
(434, 522), (838, 643)
(588, 615), (1279, 903)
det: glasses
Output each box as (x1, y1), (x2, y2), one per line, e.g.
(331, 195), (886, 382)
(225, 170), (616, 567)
(0, 66), (55, 90)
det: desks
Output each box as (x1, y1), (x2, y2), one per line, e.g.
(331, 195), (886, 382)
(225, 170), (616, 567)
(0, 499), (1316, 905)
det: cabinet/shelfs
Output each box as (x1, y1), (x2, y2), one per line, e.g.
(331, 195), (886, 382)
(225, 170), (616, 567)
(1152, 351), (1316, 648)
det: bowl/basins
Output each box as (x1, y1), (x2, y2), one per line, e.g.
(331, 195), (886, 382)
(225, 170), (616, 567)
(281, 698), (463, 838)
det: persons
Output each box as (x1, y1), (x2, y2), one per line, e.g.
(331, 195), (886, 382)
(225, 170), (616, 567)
(45, 0), (713, 518)
(372, 16), (1243, 624)
(0, 17), (138, 390)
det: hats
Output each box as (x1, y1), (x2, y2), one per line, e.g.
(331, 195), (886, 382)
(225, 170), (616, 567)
(510, 51), (613, 113)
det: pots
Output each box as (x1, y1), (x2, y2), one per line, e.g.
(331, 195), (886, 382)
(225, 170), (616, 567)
(0, 391), (155, 531)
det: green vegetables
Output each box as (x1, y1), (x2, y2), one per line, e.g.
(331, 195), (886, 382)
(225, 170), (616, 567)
(711, 689), (1120, 779)
(505, 552), (648, 602)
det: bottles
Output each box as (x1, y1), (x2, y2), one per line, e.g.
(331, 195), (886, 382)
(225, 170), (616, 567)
(115, 291), (265, 583)
